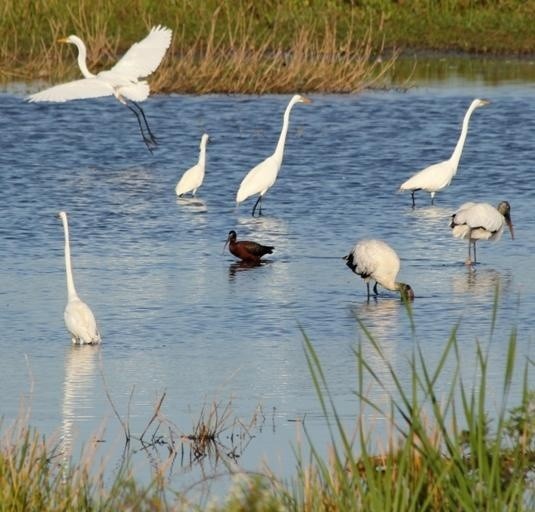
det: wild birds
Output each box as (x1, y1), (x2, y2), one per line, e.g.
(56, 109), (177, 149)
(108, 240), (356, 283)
(395, 98), (491, 207)
(175, 132), (212, 198)
(449, 201), (515, 264)
(21, 24), (173, 153)
(236, 94), (312, 218)
(342, 240), (414, 306)
(223, 231), (274, 265)
(53, 210), (102, 345)
